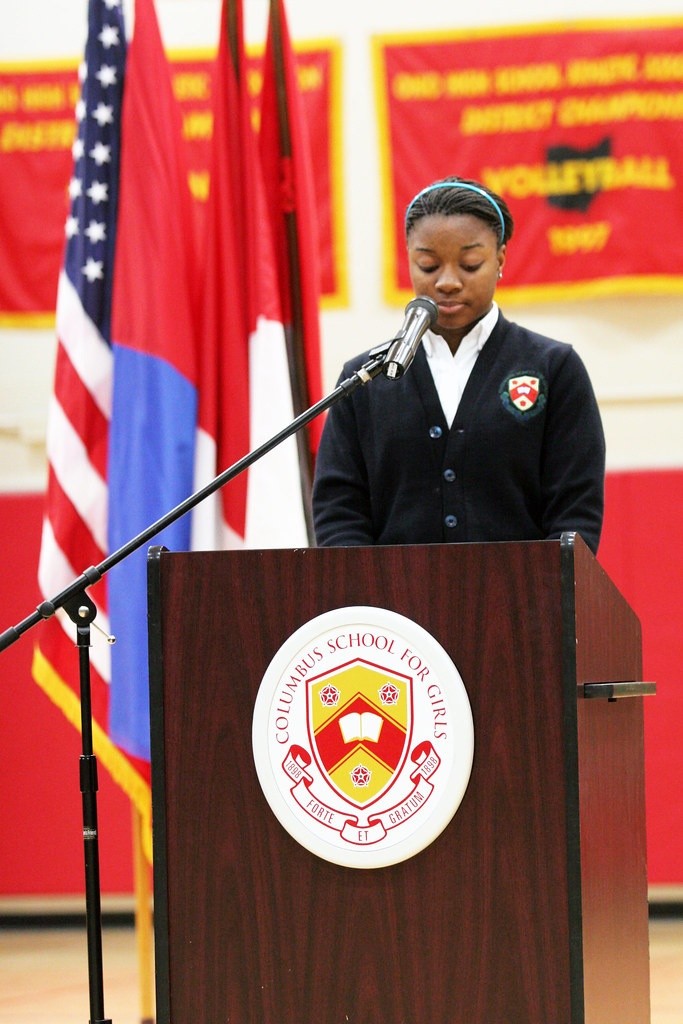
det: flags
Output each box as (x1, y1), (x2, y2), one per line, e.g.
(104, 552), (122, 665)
(30, 1), (319, 857)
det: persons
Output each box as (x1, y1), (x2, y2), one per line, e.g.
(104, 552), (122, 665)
(311, 174), (609, 554)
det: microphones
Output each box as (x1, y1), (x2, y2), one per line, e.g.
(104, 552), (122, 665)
(382, 294), (439, 380)
